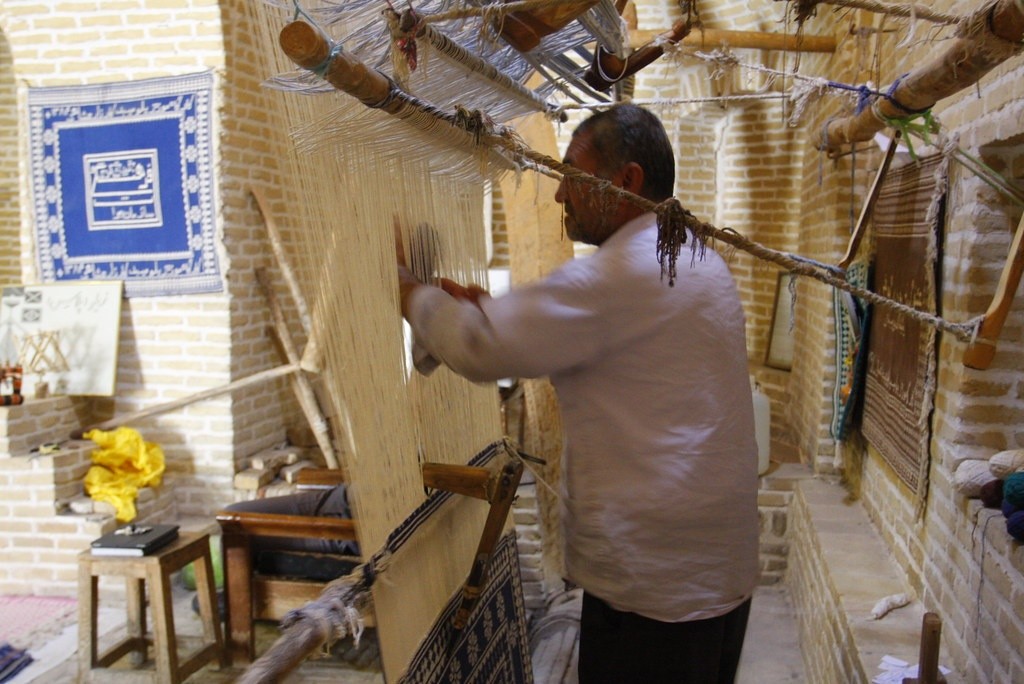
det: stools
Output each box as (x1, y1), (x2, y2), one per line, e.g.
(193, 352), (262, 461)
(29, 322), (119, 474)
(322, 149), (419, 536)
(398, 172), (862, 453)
(76, 530), (227, 684)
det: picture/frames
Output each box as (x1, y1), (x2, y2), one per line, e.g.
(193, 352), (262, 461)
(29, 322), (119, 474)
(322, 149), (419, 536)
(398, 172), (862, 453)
(763, 272), (796, 373)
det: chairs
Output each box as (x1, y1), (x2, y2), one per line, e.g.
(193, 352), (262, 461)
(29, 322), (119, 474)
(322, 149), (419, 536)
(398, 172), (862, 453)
(215, 468), (376, 666)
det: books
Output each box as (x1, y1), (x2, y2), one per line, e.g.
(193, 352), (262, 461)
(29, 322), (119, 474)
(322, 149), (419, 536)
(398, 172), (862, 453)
(91, 522), (181, 557)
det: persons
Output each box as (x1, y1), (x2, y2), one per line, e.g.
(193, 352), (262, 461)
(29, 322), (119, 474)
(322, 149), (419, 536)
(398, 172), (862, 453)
(191, 482), (362, 623)
(392, 103), (762, 684)
